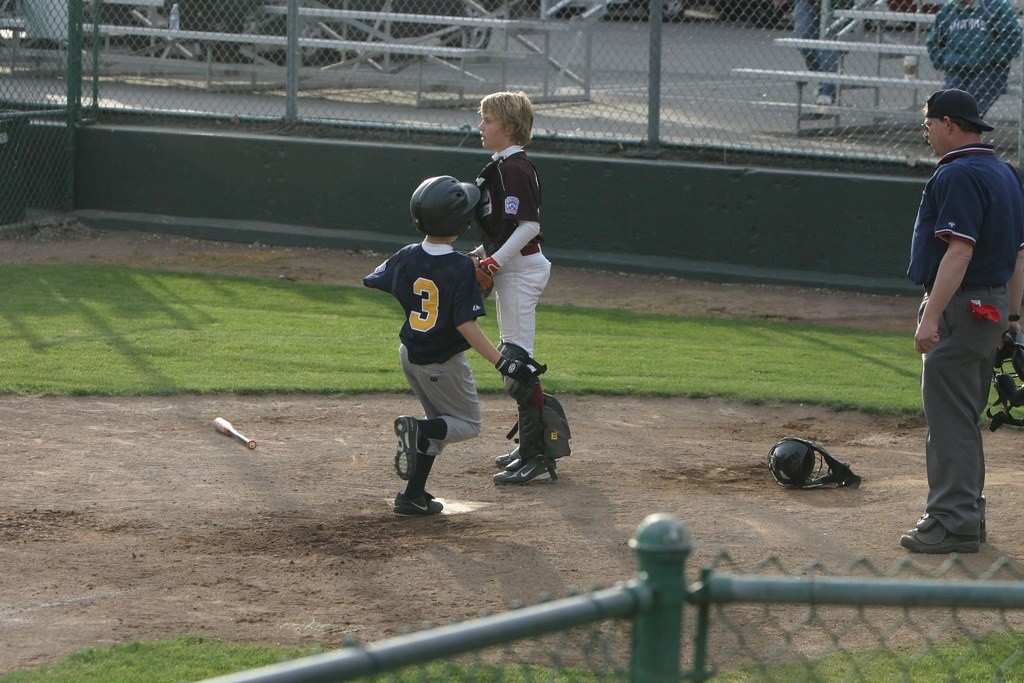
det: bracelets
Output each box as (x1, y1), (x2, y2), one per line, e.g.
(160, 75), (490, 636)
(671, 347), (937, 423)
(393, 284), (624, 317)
(1008, 313), (1021, 322)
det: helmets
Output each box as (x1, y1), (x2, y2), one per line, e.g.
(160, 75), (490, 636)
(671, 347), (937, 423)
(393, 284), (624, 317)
(410, 175), (481, 237)
(768, 437), (849, 489)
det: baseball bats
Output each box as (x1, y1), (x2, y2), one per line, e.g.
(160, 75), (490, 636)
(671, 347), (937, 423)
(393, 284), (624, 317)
(213, 416), (257, 450)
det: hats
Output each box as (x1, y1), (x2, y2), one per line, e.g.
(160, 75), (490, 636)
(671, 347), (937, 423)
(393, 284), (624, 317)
(926, 88), (994, 132)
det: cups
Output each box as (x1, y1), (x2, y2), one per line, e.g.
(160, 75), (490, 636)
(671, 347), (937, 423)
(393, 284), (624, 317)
(904, 55), (918, 81)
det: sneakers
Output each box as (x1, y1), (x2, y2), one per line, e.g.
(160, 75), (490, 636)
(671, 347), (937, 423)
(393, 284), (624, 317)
(492, 455), (557, 484)
(494, 447), (521, 467)
(393, 492), (444, 516)
(393, 416), (423, 480)
(900, 514), (986, 554)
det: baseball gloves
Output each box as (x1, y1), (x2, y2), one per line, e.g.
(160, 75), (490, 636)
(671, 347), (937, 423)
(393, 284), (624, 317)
(466, 252), (495, 300)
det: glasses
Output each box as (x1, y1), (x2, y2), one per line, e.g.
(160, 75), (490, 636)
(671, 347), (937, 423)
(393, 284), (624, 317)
(921, 119), (943, 132)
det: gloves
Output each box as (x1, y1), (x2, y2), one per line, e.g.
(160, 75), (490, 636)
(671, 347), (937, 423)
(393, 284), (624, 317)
(495, 356), (532, 386)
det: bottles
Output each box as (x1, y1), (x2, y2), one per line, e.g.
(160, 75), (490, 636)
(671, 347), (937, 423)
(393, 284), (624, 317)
(169, 3), (180, 30)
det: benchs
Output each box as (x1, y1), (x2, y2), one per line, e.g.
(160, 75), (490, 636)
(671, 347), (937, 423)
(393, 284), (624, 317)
(731, 3), (1024, 142)
(0, 0), (610, 111)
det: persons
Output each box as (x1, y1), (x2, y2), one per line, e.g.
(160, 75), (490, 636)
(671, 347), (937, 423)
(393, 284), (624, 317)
(899, 89), (1024, 556)
(363, 175), (534, 519)
(468, 91), (559, 486)
(926, 0), (1023, 122)
(794, 0), (844, 106)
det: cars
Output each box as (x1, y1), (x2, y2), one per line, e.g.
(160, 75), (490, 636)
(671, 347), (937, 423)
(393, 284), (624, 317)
(123, 0), (492, 63)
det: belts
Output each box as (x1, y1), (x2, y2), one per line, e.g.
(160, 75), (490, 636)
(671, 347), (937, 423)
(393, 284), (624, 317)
(520, 243), (539, 256)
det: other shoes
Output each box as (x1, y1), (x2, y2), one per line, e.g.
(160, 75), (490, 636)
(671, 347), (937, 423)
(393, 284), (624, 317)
(816, 96), (833, 105)
